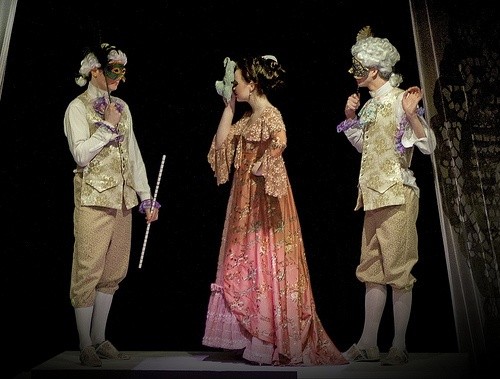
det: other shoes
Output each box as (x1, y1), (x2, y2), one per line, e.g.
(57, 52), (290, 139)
(342, 344), (380, 363)
(381, 349), (409, 366)
(96, 341), (130, 360)
(80, 345), (102, 367)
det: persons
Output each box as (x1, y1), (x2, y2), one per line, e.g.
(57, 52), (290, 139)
(336, 26), (436, 370)
(63, 44), (161, 367)
(202, 48), (350, 367)
